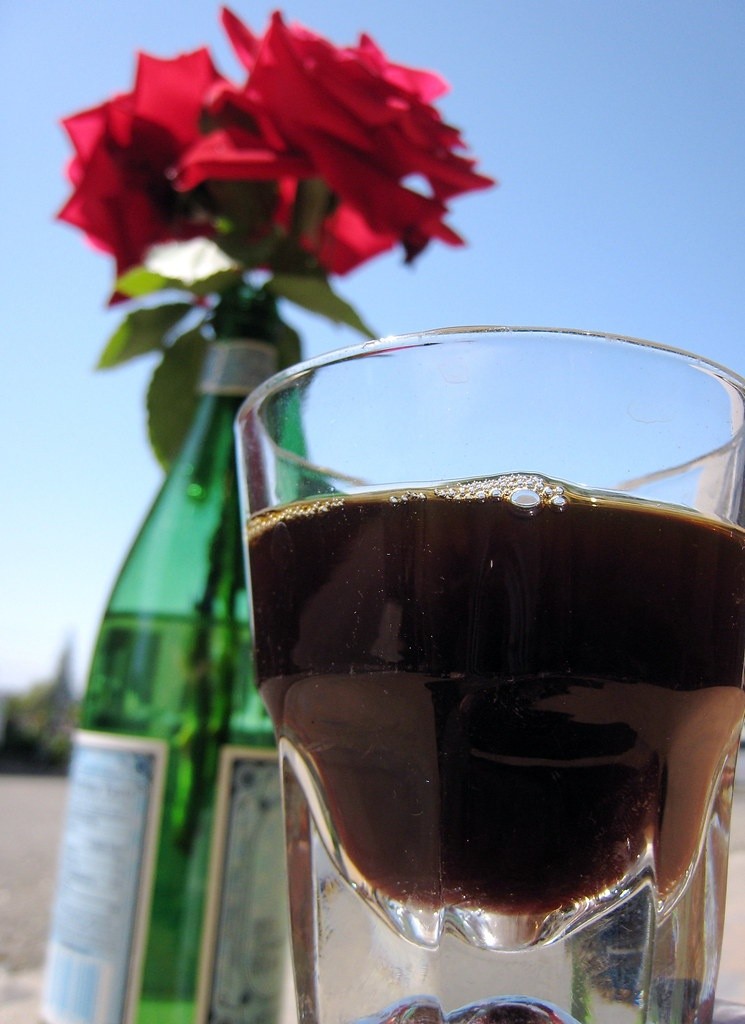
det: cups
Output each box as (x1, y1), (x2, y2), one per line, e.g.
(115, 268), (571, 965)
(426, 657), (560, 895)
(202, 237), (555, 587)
(231, 324), (744, 1024)
(39, 281), (297, 1024)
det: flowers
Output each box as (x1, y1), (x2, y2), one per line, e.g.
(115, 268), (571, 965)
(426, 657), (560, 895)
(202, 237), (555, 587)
(60, 9), (497, 869)
